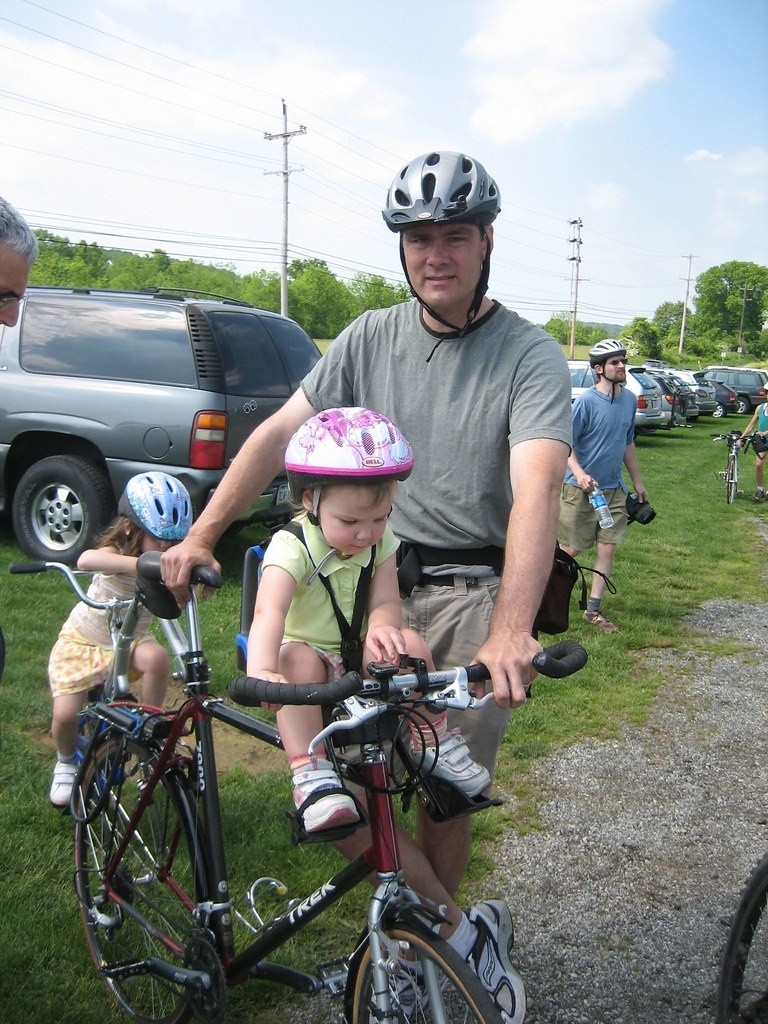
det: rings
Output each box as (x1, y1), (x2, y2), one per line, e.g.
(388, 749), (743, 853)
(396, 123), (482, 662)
(523, 681), (533, 687)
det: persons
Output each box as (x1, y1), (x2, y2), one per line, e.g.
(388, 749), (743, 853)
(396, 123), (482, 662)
(0, 195), (37, 685)
(247, 407), (492, 833)
(736, 382), (768, 502)
(557, 339), (648, 634)
(48, 471), (193, 807)
(159, 153), (574, 1024)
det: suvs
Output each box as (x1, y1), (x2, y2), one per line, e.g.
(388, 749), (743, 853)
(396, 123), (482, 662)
(660, 368), (718, 418)
(1, 286), (326, 559)
(565, 359), (666, 440)
(707, 367), (768, 415)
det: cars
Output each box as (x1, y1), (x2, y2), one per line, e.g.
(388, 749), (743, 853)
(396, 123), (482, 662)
(644, 368), (699, 428)
(705, 378), (741, 418)
(640, 360), (666, 369)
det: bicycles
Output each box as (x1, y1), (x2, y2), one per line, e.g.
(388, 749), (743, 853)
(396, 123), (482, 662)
(712, 430), (756, 505)
(7, 551), (588, 1024)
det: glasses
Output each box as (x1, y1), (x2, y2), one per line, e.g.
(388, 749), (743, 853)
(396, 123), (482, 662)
(608, 359), (628, 366)
(0, 294), (30, 312)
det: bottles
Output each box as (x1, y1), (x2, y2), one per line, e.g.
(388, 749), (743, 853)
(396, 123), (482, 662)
(588, 482), (615, 529)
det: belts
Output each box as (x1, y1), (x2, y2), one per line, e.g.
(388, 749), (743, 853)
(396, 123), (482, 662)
(414, 574), (478, 588)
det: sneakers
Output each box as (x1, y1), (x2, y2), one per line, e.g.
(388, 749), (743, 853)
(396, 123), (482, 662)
(291, 759), (360, 833)
(368, 961), (429, 1024)
(584, 610), (618, 633)
(464, 897), (528, 1024)
(414, 735), (493, 798)
(50, 760), (80, 809)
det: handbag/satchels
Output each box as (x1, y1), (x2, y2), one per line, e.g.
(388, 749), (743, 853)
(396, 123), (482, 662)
(744, 431), (767, 459)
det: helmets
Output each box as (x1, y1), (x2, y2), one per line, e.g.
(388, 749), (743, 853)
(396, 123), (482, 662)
(383, 151), (501, 233)
(588, 338), (627, 369)
(285, 407), (414, 501)
(119, 472), (193, 541)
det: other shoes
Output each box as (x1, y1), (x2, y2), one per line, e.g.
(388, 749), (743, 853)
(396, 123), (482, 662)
(752, 488), (764, 500)
